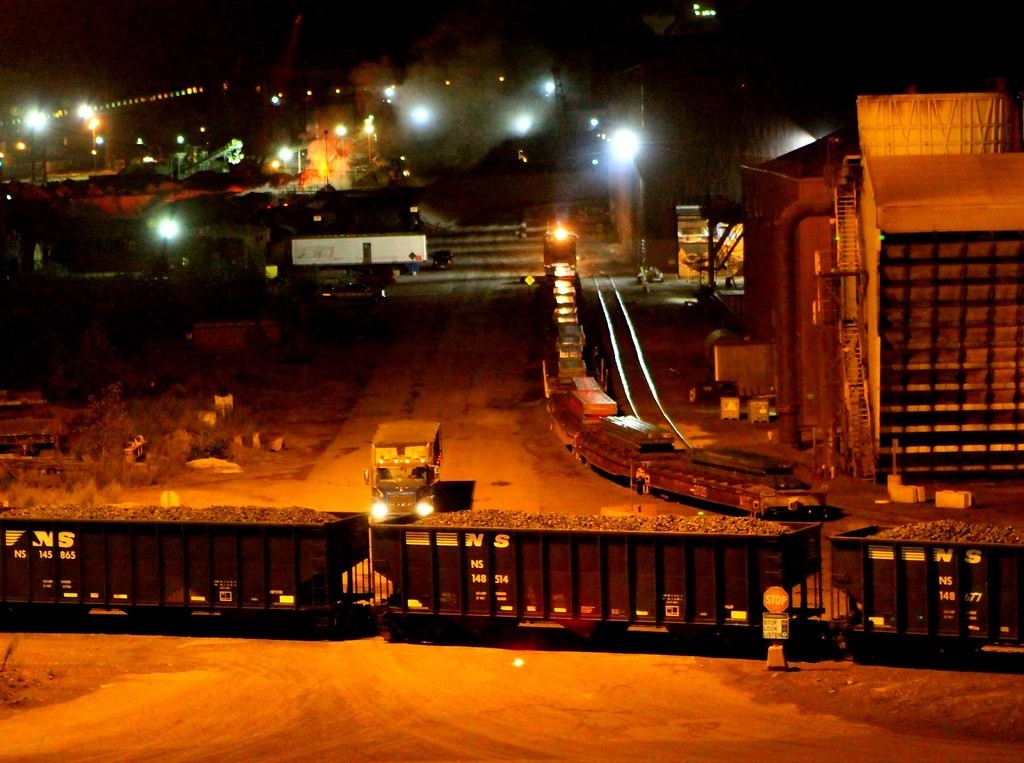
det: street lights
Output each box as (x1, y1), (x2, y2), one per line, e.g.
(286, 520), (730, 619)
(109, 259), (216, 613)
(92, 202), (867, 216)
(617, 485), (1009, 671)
(364, 125), (373, 163)
(89, 118), (100, 171)
(336, 126), (346, 159)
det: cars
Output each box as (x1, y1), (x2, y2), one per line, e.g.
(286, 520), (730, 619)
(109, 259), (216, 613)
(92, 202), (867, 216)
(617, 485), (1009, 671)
(433, 250), (452, 271)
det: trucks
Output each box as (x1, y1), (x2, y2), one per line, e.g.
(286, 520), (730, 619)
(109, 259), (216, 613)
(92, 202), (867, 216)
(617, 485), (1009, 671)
(363, 421), (442, 520)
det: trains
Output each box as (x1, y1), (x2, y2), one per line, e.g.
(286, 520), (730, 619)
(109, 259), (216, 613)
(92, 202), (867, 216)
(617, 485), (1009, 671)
(0, 505), (1024, 656)
(541, 222), (828, 524)
